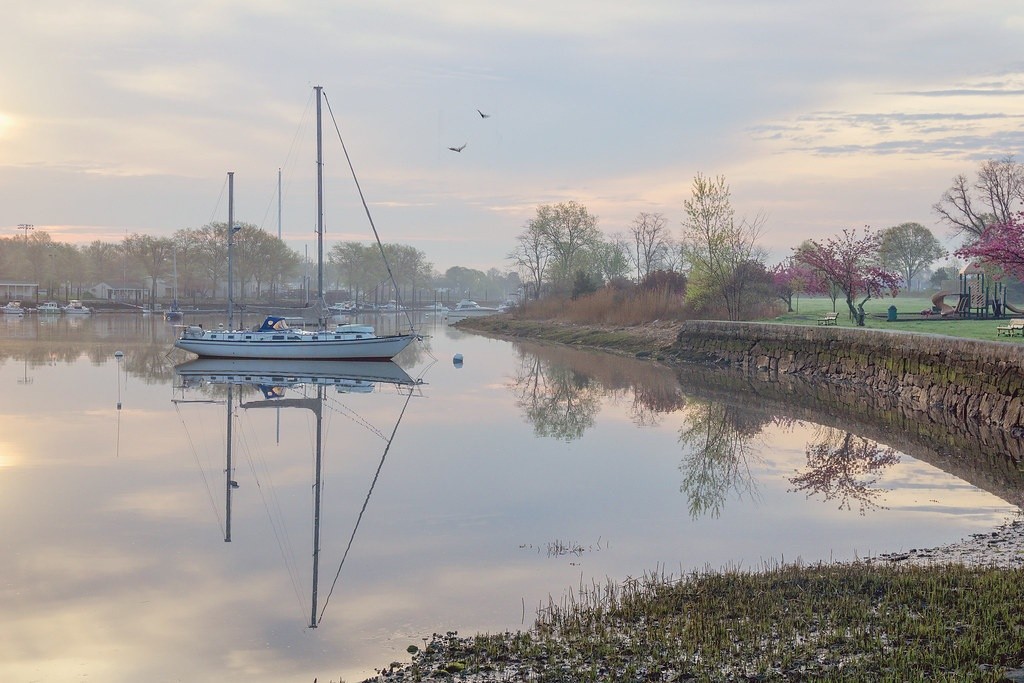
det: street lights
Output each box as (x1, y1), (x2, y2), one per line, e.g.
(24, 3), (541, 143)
(17, 224), (35, 239)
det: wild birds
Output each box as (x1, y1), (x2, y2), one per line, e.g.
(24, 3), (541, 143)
(449, 143), (467, 152)
(478, 110), (490, 118)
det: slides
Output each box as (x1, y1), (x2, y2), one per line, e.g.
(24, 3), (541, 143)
(931, 290), (955, 319)
(1002, 300), (1024, 314)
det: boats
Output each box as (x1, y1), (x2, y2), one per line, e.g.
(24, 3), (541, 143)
(330, 298), (450, 316)
(497, 293), (534, 313)
(60, 300), (92, 314)
(439, 290), (500, 323)
(0, 283), (26, 314)
(32, 302), (63, 314)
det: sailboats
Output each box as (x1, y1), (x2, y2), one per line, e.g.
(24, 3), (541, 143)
(172, 82), (425, 362)
(163, 237), (184, 322)
(167, 355), (438, 630)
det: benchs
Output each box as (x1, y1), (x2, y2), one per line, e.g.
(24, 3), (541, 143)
(996, 318), (1024, 338)
(817, 312), (839, 326)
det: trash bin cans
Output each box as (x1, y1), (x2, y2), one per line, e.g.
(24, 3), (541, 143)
(887, 305), (897, 322)
